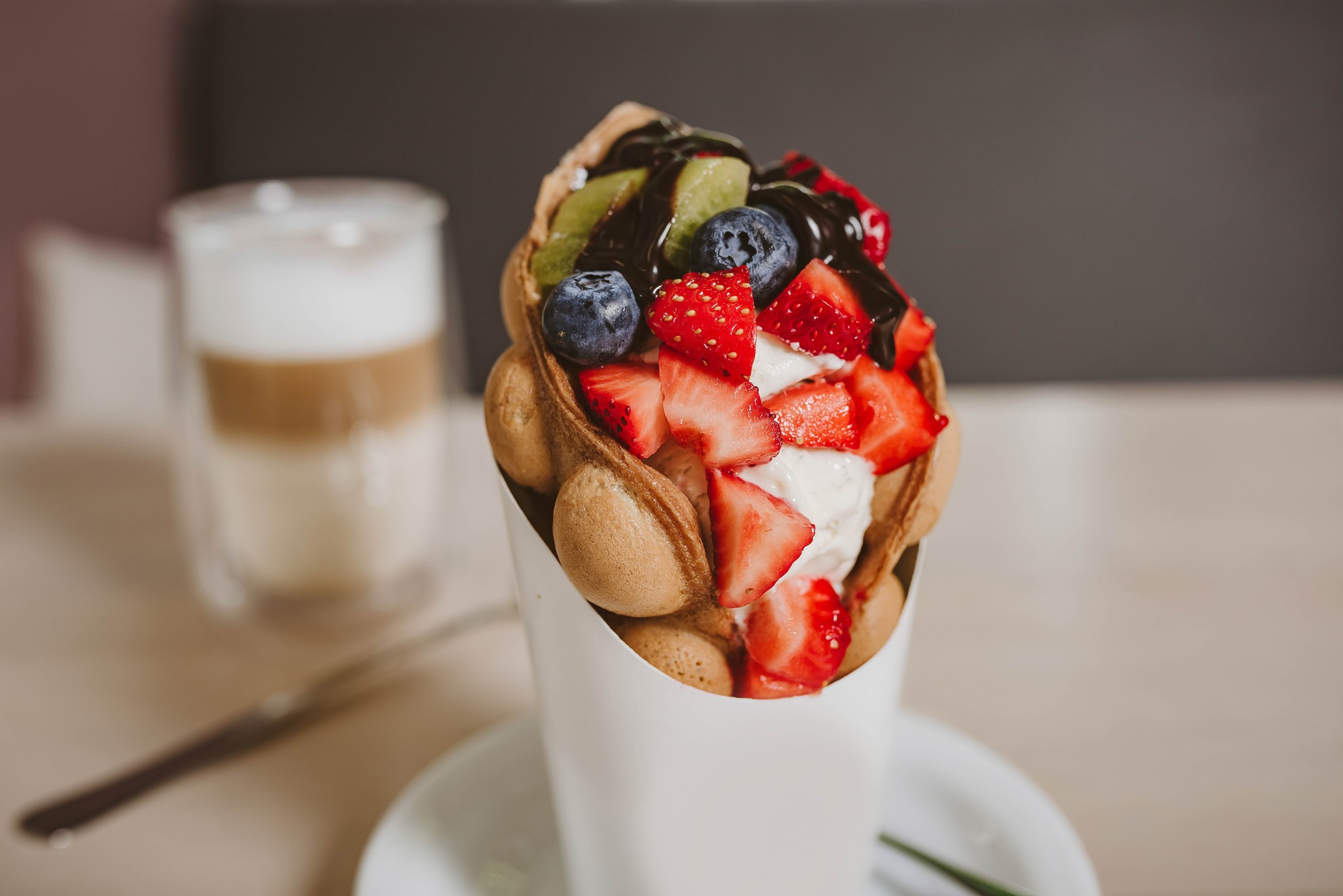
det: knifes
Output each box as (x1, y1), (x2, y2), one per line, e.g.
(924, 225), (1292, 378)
(17, 594), (515, 839)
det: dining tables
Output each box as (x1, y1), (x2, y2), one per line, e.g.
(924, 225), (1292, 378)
(0, 380), (1343, 895)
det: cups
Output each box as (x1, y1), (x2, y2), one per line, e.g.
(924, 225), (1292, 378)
(155, 177), (446, 637)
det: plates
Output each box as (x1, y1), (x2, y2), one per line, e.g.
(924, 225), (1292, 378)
(348, 701), (1106, 896)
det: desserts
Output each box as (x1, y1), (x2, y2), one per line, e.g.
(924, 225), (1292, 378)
(486, 98), (951, 698)
